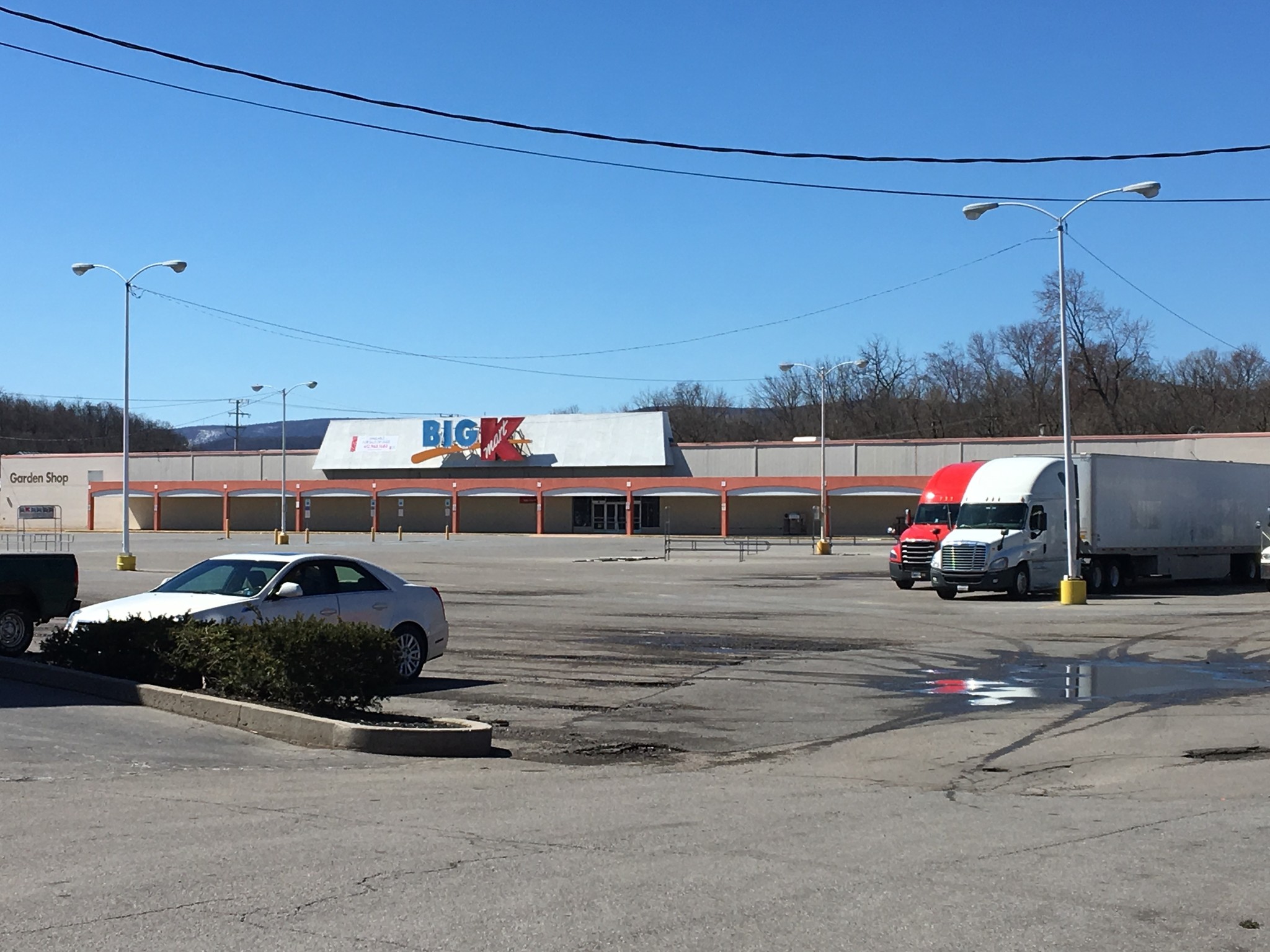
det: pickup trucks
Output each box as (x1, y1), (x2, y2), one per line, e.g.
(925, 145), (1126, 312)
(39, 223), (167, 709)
(0, 552), (81, 654)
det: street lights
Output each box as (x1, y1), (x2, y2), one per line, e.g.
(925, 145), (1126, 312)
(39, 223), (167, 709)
(964, 181), (1163, 607)
(778, 360), (869, 554)
(70, 260), (188, 570)
(248, 381), (317, 544)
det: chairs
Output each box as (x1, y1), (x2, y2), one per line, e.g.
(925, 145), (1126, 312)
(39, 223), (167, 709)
(244, 570), (267, 593)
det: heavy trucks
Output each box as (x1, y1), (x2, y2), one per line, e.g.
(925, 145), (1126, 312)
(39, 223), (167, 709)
(930, 451), (1270, 601)
(889, 458), (988, 589)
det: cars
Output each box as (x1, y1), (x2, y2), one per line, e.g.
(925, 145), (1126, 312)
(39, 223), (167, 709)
(64, 553), (449, 689)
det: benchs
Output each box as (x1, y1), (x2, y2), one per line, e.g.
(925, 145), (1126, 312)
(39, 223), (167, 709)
(339, 578), (368, 592)
